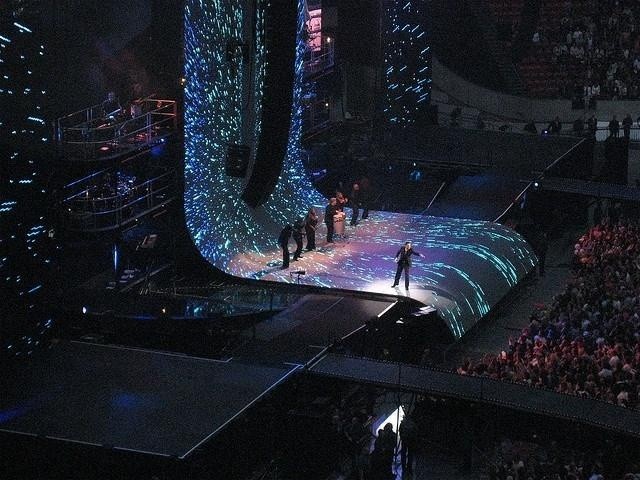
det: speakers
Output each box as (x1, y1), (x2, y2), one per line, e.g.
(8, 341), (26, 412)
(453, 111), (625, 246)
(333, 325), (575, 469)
(241, 1), (298, 207)
(224, 145), (250, 178)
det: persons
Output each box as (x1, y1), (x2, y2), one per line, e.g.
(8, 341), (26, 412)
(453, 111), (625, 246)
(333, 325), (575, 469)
(291, 213), (308, 262)
(622, 113), (634, 139)
(335, 179), (348, 212)
(325, 197), (339, 244)
(129, 83), (147, 119)
(608, 114), (620, 138)
(326, 410), (640, 480)
(390, 239), (425, 290)
(419, 347), (434, 368)
(455, 209), (640, 410)
(304, 206), (319, 251)
(99, 90), (123, 125)
(588, 114), (598, 137)
(527, 117), (538, 135)
(359, 177), (372, 220)
(547, 116), (562, 136)
(349, 182), (362, 228)
(276, 224), (293, 267)
(489, 1), (640, 100)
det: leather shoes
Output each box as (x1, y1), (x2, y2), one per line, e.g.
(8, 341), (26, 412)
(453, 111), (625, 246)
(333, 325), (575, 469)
(391, 283), (399, 287)
(293, 255), (303, 261)
(327, 239), (333, 243)
(405, 286), (408, 290)
